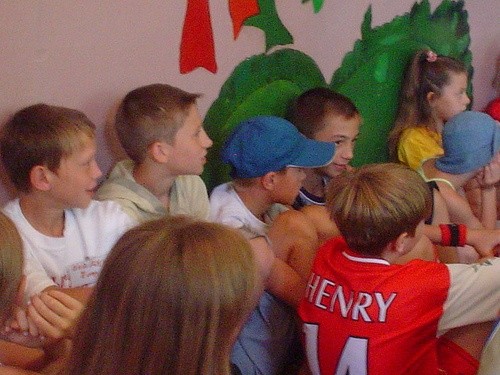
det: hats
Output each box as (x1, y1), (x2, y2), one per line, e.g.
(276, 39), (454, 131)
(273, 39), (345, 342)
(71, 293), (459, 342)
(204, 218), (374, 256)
(222, 117), (337, 178)
(435, 110), (499, 175)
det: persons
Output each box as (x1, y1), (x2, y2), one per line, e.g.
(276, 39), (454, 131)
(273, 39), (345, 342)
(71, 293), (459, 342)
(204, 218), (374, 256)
(209, 116), (341, 374)
(74, 216), (258, 375)
(275, 87), (361, 212)
(300, 163), (500, 375)
(0, 102), (139, 308)
(95, 86), (213, 227)
(417, 112), (499, 258)
(0, 217), (82, 375)
(393, 51), (472, 172)
